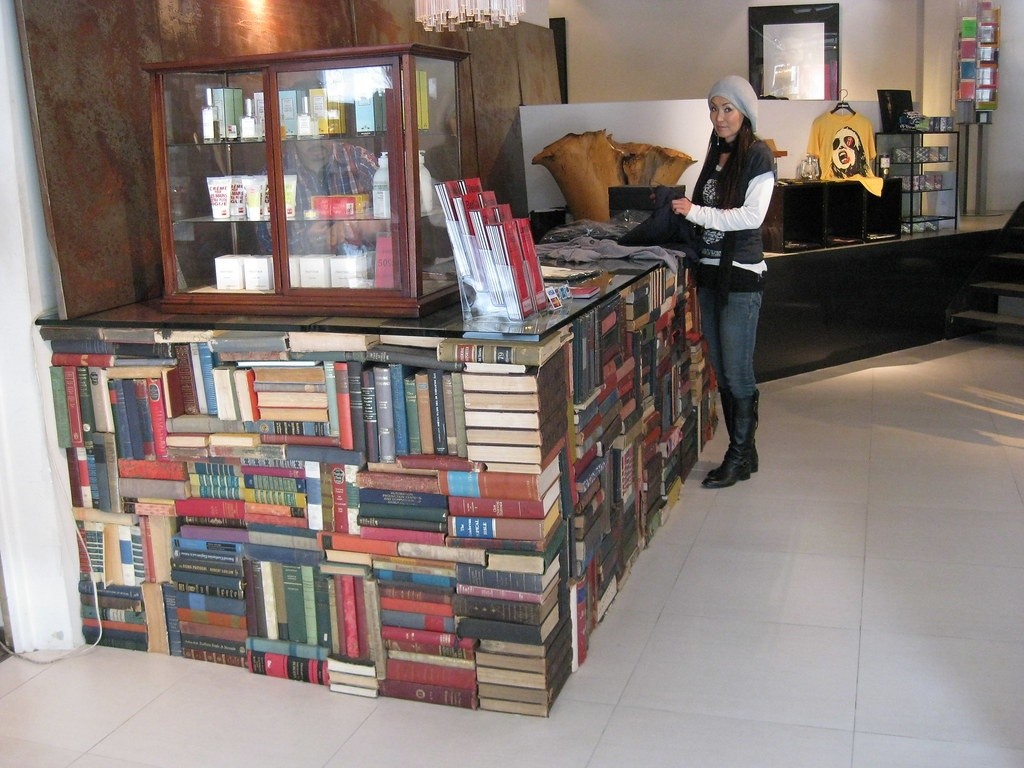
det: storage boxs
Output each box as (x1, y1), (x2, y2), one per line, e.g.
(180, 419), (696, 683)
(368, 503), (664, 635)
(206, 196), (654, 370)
(213, 255), (245, 290)
(893, 113), (952, 234)
(353, 71), (428, 133)
(330, 254), (368, 287)
(310, 195), (370, 217)
(203, 82), (346, 140)
(298, 254), (335, 288)
(956, 0), (998, 108)
(244, 255), (273, 290)
(270, 255), (304, 290)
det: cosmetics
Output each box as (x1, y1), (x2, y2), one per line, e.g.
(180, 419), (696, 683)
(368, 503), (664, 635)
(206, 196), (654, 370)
(207, 174), (298, 219)
(201, 85), (346, 142)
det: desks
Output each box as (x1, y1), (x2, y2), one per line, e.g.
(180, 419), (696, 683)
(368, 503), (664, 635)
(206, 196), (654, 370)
(698, 210), (1015, 391)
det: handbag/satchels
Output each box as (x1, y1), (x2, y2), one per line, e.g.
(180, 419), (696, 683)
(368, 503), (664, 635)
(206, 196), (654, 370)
(607, 185), (686, 223)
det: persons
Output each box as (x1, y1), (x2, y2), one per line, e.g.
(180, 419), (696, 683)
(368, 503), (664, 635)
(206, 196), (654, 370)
(649, 75), (778, 489)
(250, 80), (380, 281)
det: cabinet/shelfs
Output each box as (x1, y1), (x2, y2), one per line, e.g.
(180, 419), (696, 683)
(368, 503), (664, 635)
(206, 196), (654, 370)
(140, 45), (472, 320)
(875, 130), (962, 236)
(761, 178), (903, 253)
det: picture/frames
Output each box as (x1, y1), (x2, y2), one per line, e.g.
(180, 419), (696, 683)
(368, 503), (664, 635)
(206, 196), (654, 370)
(877, 90), (913, 131)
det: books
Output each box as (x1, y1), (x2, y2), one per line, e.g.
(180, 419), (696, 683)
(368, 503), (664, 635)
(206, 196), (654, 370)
(42, 324), (575, 718)
(543, 266), (600, 299)
(434, 176), (545, 320)
(559, 253), (719, 672)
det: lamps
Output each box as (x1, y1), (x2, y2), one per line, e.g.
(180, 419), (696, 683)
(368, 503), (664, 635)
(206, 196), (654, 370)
(415, 0), (526, 33)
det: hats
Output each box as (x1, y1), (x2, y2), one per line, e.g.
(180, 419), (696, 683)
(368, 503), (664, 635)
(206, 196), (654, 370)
(708, 76), (757, 133)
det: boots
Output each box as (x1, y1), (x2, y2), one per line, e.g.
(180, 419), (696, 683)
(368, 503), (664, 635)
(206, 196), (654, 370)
(702, 389), (760, 488)
(707, 387), (758, 476)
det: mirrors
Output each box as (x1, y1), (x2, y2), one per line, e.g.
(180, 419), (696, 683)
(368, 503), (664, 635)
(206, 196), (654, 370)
(748, 2), (841, 100)
